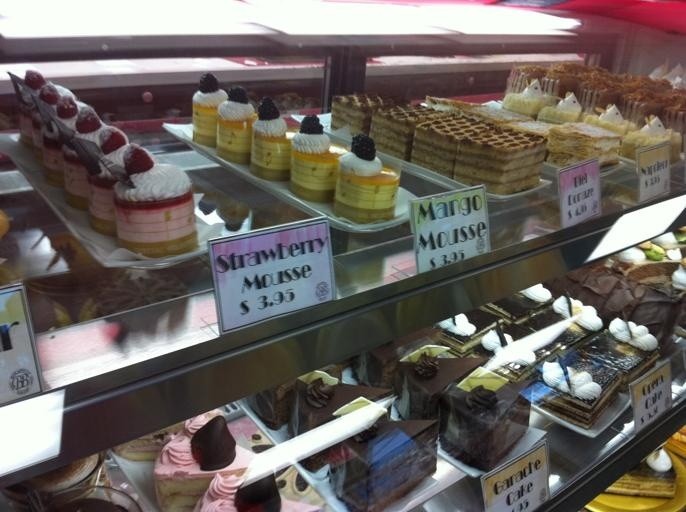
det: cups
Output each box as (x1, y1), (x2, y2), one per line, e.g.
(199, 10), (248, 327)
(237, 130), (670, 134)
(0, 449), (142, 511)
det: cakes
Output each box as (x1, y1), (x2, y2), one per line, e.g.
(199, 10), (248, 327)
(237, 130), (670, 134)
(217, 86), (258, 165)
(248, 97), (291, 180)
(7, 70), (197, 257)
(333, 133), (399, 225)
(193, 72), (229, 148)
(289, 114), (347, 203)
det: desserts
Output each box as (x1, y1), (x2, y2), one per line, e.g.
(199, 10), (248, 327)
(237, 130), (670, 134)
(0, 260), (686, 512)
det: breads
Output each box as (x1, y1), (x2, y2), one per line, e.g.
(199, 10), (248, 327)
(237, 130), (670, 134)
(453, 126), (549, 194)
(330, 90), (408, 134)
(423, 95), (485, 110)
(472, 62), (686, 169)
(410, 112), (502, 179)
(370, 103), (450, 160)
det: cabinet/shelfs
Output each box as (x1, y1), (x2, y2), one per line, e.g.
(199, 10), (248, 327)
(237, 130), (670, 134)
(0, 3), (686, 512)
